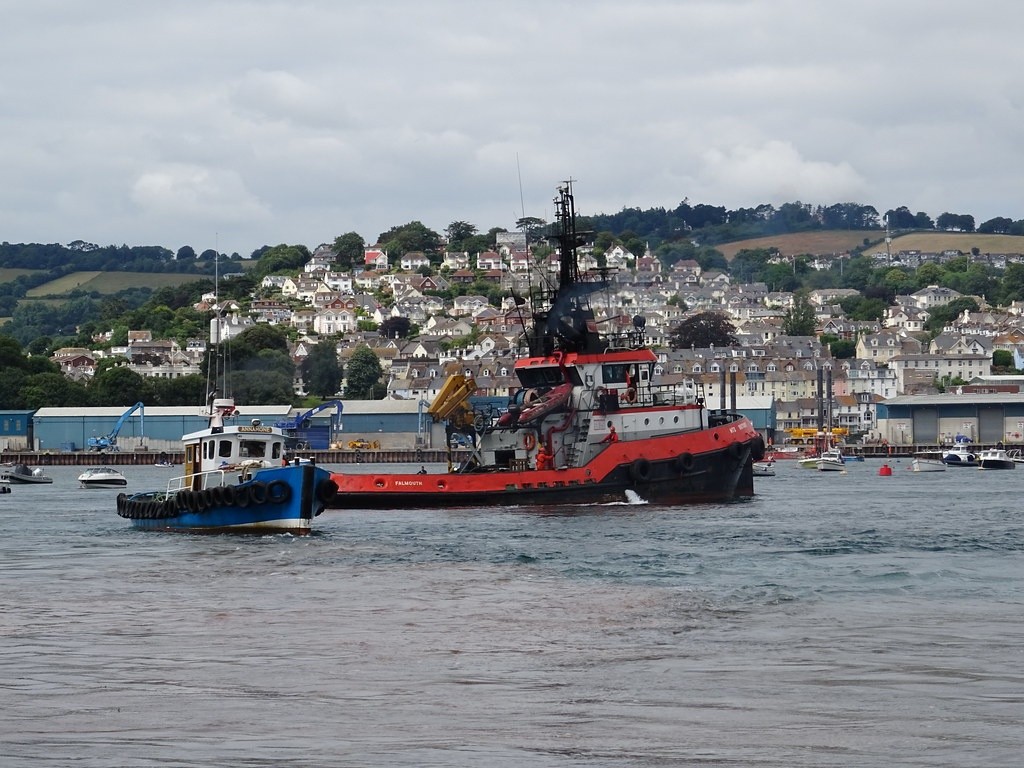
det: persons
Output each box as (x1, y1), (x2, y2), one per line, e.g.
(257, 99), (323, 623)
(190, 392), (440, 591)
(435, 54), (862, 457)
(601, 427), (618, 444)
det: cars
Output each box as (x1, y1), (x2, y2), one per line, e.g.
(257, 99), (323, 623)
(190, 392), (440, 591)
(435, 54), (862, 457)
(956, 435), (973, 444)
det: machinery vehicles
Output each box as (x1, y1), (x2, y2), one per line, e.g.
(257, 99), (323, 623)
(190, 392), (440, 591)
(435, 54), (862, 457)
(347, 438), (381, 450)
(414, 399), (475, 448)
(271, 400), (344, 450)
(87, 402), (148, 452)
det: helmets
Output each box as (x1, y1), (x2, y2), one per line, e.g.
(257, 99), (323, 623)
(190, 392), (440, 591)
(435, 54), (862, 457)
(539, 447), (545, 451)
(610, 426), (615, 430)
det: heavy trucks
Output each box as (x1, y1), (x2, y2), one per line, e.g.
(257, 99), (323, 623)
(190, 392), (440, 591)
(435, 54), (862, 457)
(784, 428), (847, 444)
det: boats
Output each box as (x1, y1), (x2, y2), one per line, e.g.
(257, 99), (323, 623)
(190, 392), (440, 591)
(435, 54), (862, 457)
(154, 462), (174, 467)
(798, 432), (867, 471)
(115, 397), (341, 538)
(751, 462), (776, 477)
(0, 479), (11, 494)
(912, 457), (948, 471)
(1006, 449), (1024, 464)
(325, 176), (768, 508)
(942, 442), (981, 466)
(1, 464), (54, 484)
(978, 447), (1015, 469)
(78, 466), (127, 488)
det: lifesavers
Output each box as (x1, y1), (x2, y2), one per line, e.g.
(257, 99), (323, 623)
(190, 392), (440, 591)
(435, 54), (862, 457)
(624, 388), (637, 404)
(521, 433), (535, 450)
(593, 385), (607, 403)
